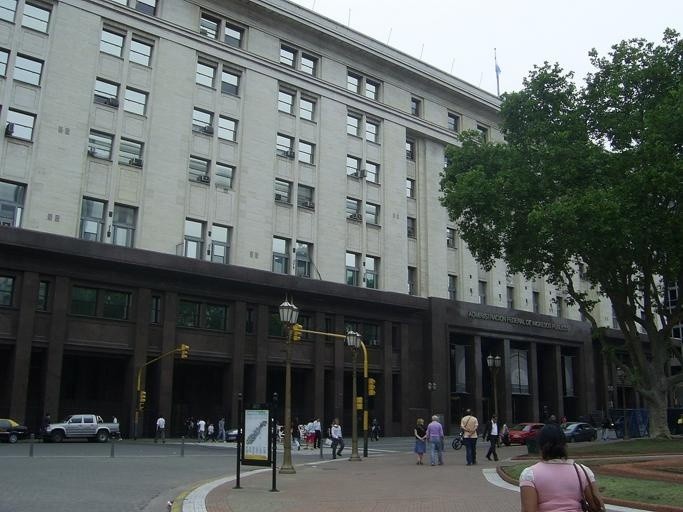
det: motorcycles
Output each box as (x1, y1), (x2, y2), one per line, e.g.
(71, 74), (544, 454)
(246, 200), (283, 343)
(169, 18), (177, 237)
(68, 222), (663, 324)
(451, 432), (464, 450)
(499, 433), (512, 445)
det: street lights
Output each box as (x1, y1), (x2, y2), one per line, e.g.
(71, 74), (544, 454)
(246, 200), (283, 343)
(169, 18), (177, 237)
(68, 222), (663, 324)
(277, 293), (298, 470)
(347, 330), (361, 458)
(485, 354), (501, 423)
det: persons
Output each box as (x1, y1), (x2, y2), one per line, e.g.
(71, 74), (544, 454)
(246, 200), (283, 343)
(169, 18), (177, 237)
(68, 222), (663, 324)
(112, 415), (123, 440)
(519, 422), (605, 512)
(414, 418), (427, 465)
(460, 408), (479, 466)
(426, 415), (445, 466)
(502, 414), (611, 446)
(182, 414), (227, 444)
(154, 414), (166, 443)
(39, 413), (51, 442)
(370, 418), (380, 441)
(291, 416), (344, 459)
(482, 414), (500, 461)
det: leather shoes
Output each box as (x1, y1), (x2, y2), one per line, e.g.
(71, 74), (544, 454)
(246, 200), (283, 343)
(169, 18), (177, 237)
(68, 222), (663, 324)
(333, 457), (336, 459)
(336, 452), (342, 456)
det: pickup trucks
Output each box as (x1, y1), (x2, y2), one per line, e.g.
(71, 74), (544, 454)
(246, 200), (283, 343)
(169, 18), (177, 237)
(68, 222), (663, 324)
(43, 414), (118, 443)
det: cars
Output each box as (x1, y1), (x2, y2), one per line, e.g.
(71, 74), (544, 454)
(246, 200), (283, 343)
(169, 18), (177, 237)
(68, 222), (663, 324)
(223, 427), (243, 441)
(0, 419), (27, 443)
(508, 422), (596, 444)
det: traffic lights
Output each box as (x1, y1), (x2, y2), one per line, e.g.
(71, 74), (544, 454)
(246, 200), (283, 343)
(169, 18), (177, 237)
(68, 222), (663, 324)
(181, 344), (189, 359)
(139, 403), (144, 414)
(140, 391), (146, 404)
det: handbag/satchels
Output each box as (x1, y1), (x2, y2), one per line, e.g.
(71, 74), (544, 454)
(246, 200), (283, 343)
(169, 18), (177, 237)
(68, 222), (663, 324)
(580, 480), (606, 511)
(325, 438), (334, 446)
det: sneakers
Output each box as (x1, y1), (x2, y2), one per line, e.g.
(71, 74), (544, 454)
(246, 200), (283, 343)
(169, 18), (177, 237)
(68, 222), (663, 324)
(465, 454), (500, 466)
(416, 460), (444, 466)
